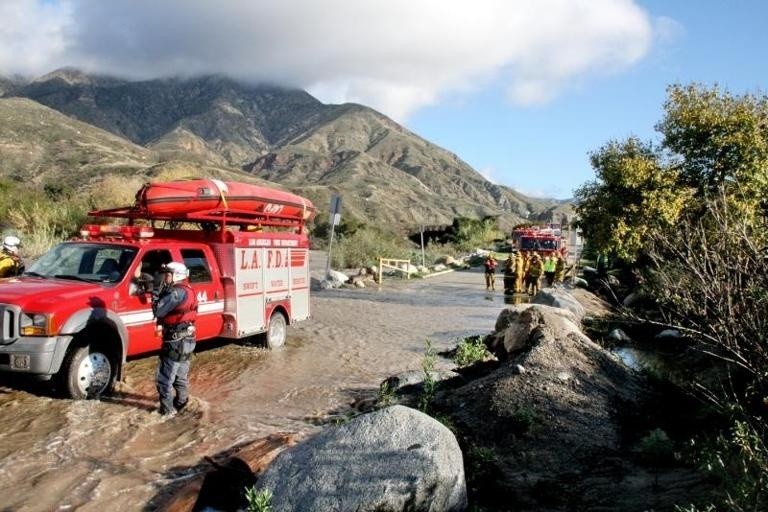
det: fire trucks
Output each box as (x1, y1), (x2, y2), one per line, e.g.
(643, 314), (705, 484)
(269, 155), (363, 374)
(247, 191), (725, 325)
(511, 220), (566, 276)
(0, 175), (321, 403)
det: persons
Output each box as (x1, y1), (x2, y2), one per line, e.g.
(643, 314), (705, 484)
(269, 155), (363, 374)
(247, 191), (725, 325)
(0, 236), (24, 277)
(501, 248), (568, 297)
(483, 253), (497, 290)
(126, 260), (154, 292)
(152, 262), (197, 415)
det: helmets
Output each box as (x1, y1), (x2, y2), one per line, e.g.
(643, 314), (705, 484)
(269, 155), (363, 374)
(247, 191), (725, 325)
(3, 236), (24, 250)
(160, 261), (189, 285)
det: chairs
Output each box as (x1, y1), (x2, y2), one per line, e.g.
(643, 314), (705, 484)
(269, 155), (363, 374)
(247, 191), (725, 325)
(96, 258), (117, 275)
